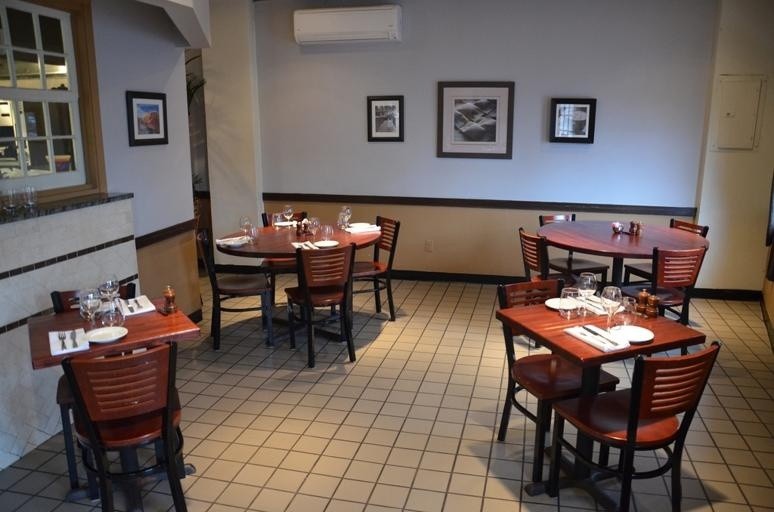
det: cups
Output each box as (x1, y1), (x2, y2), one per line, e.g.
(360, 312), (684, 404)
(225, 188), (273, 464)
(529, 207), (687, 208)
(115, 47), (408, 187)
(2, 186), (38, 211)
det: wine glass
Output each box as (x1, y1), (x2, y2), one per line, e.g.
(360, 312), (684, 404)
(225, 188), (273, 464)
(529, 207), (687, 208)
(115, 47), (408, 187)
(238, 204), (352, 241)
(78, 274), (122, 327)
(560, 274), (637, 327)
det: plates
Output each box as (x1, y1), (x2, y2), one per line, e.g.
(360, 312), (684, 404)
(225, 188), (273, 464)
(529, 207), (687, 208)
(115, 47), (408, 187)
(545, 298), (581, 310)
(85, 326), (129, 344)
(314, 241), (340, 247)
(610, 325), (654, 343)
(225, 241), (248, 247)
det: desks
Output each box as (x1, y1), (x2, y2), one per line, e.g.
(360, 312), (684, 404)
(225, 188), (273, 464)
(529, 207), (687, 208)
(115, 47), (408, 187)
(216, 225), (380, 341)
(28, 299), (201, 512)
(496, 303), (706, 512)
(537, 220), (709, 289)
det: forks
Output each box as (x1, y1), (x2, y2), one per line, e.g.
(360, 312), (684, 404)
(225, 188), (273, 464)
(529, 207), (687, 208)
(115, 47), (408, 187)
(135, 300), (142, 311)
(56, 328), (67, 350)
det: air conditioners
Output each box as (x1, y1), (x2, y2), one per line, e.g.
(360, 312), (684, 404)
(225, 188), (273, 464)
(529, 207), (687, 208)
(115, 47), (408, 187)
(293, 4), (402, 44)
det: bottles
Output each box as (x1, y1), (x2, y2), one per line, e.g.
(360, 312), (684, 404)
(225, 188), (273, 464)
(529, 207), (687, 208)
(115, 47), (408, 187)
(164, 287), (177, 310)
(629, 219), (642, 235)
(639, 288), (658, 317)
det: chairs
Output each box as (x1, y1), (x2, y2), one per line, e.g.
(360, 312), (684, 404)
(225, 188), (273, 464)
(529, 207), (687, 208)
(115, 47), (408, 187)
(518, 227), (576, 348)
(197, 231), (273, 349)
(624, 219), (710, 283)
(621, 247), (705, 355)
(539, 213), (609, 288)
(285, 243), (356, 368)
(352, 216), (401, 321)
(51, 283), (136, 490)
(262, 211), (308, 306)
(548, 340), (722, 511)
(498, 279), (619, 484)
(61, 340), (188, 512)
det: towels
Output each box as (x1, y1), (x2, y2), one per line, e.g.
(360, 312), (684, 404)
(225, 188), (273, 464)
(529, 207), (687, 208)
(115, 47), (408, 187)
(115, 295), (155, 317)
(216, 235), (249, 245)
(565, 325), (626, 352)
(580, 297), (623, 315)
(292, 241), (319, 249)
(344, 225), (382, 232)
(48, 328), (89, 357)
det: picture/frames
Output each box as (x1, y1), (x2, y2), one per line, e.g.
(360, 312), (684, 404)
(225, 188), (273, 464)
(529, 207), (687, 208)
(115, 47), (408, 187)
(549, 98), (597, 144)
(437, 80), (515, 159)
(367, 95), (404, 142)
(126, 90), (168, 146)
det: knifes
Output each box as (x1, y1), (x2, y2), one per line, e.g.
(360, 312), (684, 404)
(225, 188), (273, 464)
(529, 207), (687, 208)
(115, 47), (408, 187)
(70, 328), (79, 348)
(580, 325), (618, 347)
(124, 298), (134, 314)
